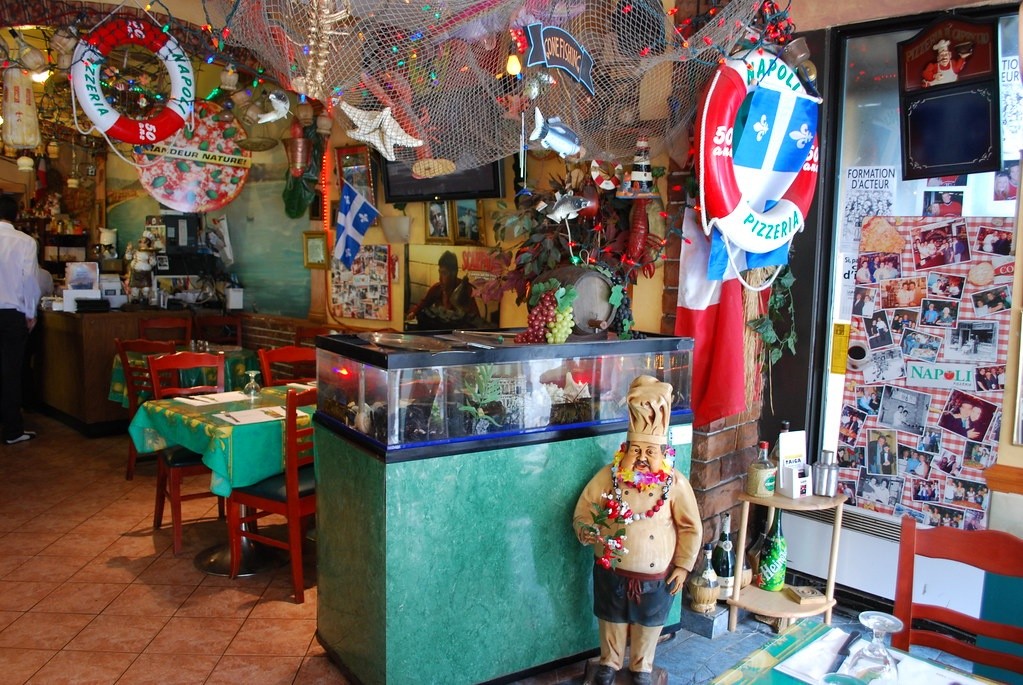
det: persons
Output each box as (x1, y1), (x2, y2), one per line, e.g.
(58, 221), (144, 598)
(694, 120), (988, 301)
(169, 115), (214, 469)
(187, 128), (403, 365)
(428, 201), (448, 239)
(0, 192), (55, 444)
(458, 207), (476, 237)
(125, 230), (156, 306)
(406, 251), (489, 327)
(921, 37), (973, 86)
(574, 374), (702, 685)
(834, 161), (1019, 532)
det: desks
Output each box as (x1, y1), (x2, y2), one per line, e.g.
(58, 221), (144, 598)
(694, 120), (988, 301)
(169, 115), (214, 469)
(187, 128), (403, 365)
(129, 381), (316, 577)
(109, 344), (260, 468)
(710, 614), (1001, 685)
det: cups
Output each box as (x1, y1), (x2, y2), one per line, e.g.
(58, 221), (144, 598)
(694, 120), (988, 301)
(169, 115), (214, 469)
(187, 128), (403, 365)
(819, 673), (867, 685)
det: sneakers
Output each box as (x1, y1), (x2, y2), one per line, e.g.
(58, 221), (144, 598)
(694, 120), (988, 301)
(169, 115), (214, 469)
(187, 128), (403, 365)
(7, 430), (37, 445)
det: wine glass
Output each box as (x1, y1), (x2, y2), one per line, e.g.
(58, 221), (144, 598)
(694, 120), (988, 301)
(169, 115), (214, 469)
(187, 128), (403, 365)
(244, 371), (261, 396)
(846, 611), (903, 685)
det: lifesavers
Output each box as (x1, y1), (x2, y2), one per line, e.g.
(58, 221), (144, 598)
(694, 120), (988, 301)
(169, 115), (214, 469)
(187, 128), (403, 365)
(70, 17), (196, 146)
(693, 48), (820, 254)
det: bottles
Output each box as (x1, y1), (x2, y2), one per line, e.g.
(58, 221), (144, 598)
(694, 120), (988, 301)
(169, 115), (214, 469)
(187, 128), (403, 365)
(757, 507), (788, 592)
(770, 421), (790, 466)
(690, 543), (720, 613)
(711, 512), (735, 597)
(748, 521), (769, 582)
(735, 530), (752, 589)
(812, 449), (839, 497)
(745, 440), (776, 498)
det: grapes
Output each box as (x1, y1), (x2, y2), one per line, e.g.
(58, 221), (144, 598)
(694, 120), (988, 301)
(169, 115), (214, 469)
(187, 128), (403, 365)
(514, 292), (575, 344)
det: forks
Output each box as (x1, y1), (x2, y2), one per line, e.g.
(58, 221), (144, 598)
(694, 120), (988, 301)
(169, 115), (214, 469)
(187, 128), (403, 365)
(224, 412), (240, 423)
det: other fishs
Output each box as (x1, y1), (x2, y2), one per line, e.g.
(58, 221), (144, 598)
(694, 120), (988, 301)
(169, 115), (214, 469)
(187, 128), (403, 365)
(528, 105), (581, 160)
(545, 194), (591, 224)
(256, 89), (291, 125)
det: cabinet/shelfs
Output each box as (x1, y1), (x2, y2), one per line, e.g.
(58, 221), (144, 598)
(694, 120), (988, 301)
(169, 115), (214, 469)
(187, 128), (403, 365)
(727, 486), (849, 634)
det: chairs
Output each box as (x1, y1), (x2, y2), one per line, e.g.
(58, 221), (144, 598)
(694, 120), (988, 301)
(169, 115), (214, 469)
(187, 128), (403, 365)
(138, 315), (194, 347)
(294, 327), (345, 379)
(148, 351), (250, 555)
(114, 334), (200, 481)
(227, 388), (320, 604)
(890, 517), (1023, 677)
(258, 346), (320, 387)
(187, 314), (242, 346)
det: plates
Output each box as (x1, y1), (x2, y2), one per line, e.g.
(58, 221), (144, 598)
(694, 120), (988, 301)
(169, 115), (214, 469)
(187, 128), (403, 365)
(356, 331), (452, 352)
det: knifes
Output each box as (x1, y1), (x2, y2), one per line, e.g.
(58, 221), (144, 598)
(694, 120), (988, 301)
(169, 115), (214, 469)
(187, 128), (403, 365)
(179, 395), (210, 403)
(827, 629), (861, 676)
(280, 406), (298, 416)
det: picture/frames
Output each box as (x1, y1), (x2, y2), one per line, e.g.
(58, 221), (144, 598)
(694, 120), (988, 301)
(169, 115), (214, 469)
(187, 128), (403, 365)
(424, 199), (455, 246)
(333, 142), (378, 226)
(451, 198), (486, 247)
(302, 231), (329, 270)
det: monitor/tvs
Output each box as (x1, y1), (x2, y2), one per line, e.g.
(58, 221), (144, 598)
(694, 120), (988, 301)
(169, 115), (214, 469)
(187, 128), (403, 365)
(380, 149), (506, 204)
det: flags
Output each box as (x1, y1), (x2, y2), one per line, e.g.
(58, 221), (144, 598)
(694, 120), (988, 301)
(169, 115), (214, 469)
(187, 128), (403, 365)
(706, 85), (818, 280)
(672, 195), (747, 430)
(333, 182), (378, 271)
